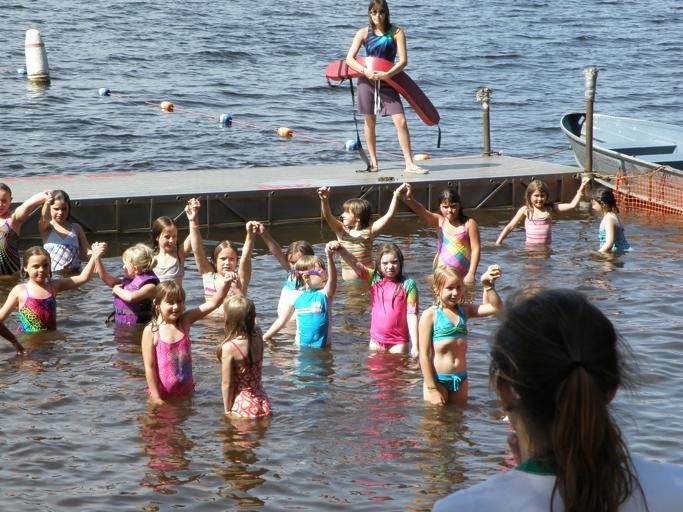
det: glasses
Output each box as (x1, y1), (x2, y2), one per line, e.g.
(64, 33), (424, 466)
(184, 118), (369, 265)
(371, 9), (385, 15)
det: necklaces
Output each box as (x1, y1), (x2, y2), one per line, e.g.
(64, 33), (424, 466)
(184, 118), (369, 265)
(374, 27), (385, 35)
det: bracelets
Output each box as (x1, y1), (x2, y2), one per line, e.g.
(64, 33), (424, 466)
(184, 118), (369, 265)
(43, 201), (50, 209)
(425, 384), (439, 390)
(392, 190), (403, 200)
(229, 283), (240, 289)
(483, 286), (493, 291)
(359, 64), (365, 75)
(575, 189), (585, 197)
(467, 270), (476, 276)
(188, 222), (200, 229)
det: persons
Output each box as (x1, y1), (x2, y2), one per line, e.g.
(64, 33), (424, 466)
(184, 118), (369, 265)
(38, 189), (107, 276)
(0, 241), (107, 333)
(417, 263), (503, 410)
(148, 196), (201, 289)
(181, 200), (259, 317)
(495, 175), (591, 246)
(324, 239), (419, 361)
(0, 182), (52, 276)
(215, 270), (271, 422)
(316, 182), (409, 281)
(478, 262), (547, 318)
(429, 285), (681, 512)
(344, 0), (429, 174)
(587, 186), (633, 252)
(89, 241), (162, 327)
(140, 270), (238, 405)
(398, 182), (481, 284)
(260, 239), (341, 350)
(0, 318), (26, 357)
(250, 219), (316, 321)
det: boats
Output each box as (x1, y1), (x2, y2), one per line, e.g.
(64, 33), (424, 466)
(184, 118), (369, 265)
(558, 111), (682, 213)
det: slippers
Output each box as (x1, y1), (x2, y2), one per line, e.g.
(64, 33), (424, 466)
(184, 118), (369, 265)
(369, 160), (378, 172)
(406, 165), (429, 174)
